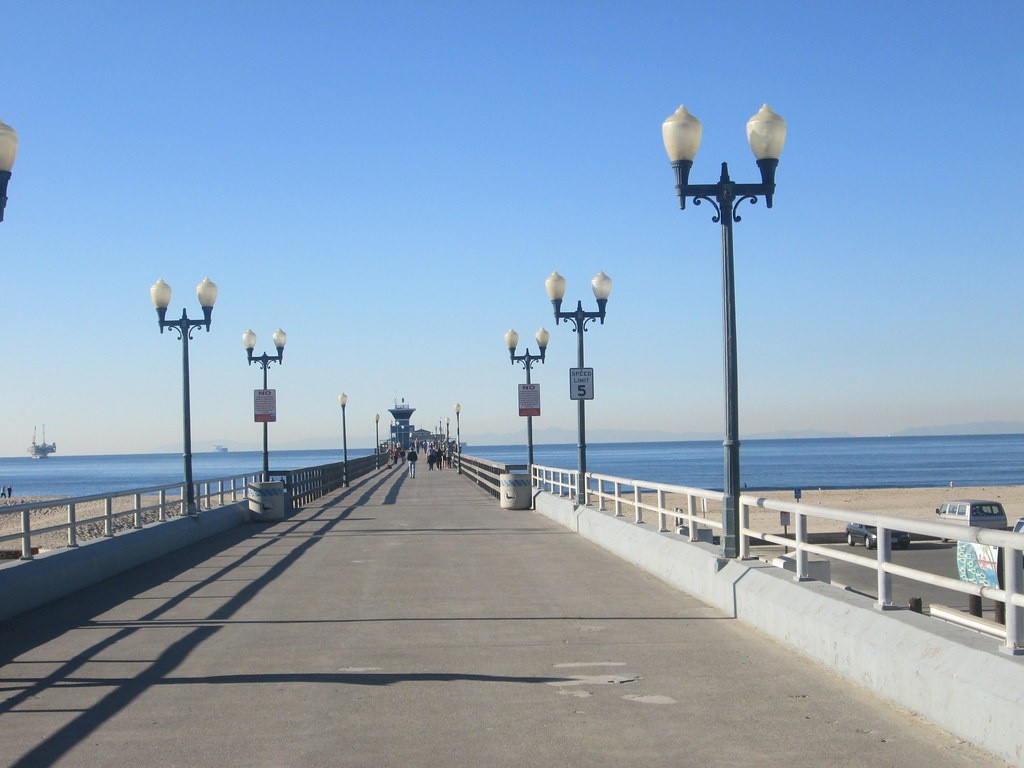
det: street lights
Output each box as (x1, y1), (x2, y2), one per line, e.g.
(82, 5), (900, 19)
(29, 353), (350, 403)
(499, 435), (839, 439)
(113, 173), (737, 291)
(453, 402), (462, 474)
(339, 392), (349, 487)
(504, 328), (549, 486)
(545, 270), (612, 506)
(390, 420), (393, 460)
(242, 328), (287, 482)
(445, 417), (450, 443)
(151, 276), (218, 515)
(661, 105), (788, 559)
(375, 413), (380, 470)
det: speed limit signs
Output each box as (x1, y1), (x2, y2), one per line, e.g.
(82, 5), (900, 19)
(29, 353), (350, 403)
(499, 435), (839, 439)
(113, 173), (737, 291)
(569, 367), (594, 400)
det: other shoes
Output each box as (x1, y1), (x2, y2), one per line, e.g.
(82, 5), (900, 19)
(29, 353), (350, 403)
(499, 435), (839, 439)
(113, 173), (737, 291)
(410, 476), (412, 478)
(412, 477), (415, 478)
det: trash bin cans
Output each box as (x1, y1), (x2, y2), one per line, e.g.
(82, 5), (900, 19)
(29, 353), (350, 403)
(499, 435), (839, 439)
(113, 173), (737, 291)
(249, 481), (284, 522)
(500, 474), (532, 509)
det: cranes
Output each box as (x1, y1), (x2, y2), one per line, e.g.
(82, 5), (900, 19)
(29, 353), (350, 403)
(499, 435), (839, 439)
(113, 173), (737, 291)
(32, 425), (36, 446)
(42, 424), (46, 445)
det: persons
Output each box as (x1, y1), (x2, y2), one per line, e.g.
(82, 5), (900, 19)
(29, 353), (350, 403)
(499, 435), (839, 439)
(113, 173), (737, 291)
(407, 447), (417, 478)
(386, 443), (405, 465)
(0, 486), (6, 498)
(7, 486), (12, 498)
(412, 438), (450, 471)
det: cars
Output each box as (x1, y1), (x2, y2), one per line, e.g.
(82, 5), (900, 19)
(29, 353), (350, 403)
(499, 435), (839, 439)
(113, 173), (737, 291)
(846, 522), (910, 550)
(1012, 517), (1024, 568)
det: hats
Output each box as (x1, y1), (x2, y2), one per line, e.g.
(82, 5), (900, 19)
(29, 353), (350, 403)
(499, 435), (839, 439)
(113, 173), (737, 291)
(409, 447), (414, 450)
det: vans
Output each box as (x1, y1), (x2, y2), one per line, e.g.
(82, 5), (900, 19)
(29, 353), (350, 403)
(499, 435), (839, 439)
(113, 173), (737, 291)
(936, 499), (1007, 543)
